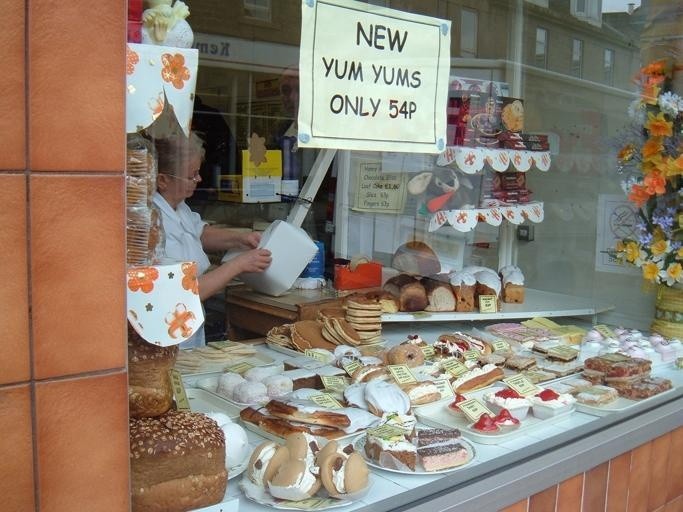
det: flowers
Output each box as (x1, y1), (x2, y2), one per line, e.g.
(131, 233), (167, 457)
(599, 57), (683, 290)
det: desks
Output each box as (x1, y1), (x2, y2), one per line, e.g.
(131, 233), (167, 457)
(165, 327), (683, 511)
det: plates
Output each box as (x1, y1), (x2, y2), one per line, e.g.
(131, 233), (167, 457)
(224, 445), (254, 481)
(240, 468), (374, 512)
(352, 427), (477, 475)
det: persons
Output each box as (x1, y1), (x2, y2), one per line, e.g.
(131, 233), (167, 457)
(147, 127), (272, 351)
(190, 94), (242, 199)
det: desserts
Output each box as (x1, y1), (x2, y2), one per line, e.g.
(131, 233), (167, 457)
(219, 317), (683, 495)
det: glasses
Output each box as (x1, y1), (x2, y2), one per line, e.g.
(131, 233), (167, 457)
(162, 173), (201, 184)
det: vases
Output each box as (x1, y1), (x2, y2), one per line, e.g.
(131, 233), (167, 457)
(649, 284), (683, 344)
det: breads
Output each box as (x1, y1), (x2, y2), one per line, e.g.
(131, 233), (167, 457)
(129, 409), (228, 512)
(366, 265), (525, 313)
(392, 241), (441, 274)
(128, 321), (179, 418)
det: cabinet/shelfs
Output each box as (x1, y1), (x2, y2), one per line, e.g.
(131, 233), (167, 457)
(217, 280), (347, 346)
